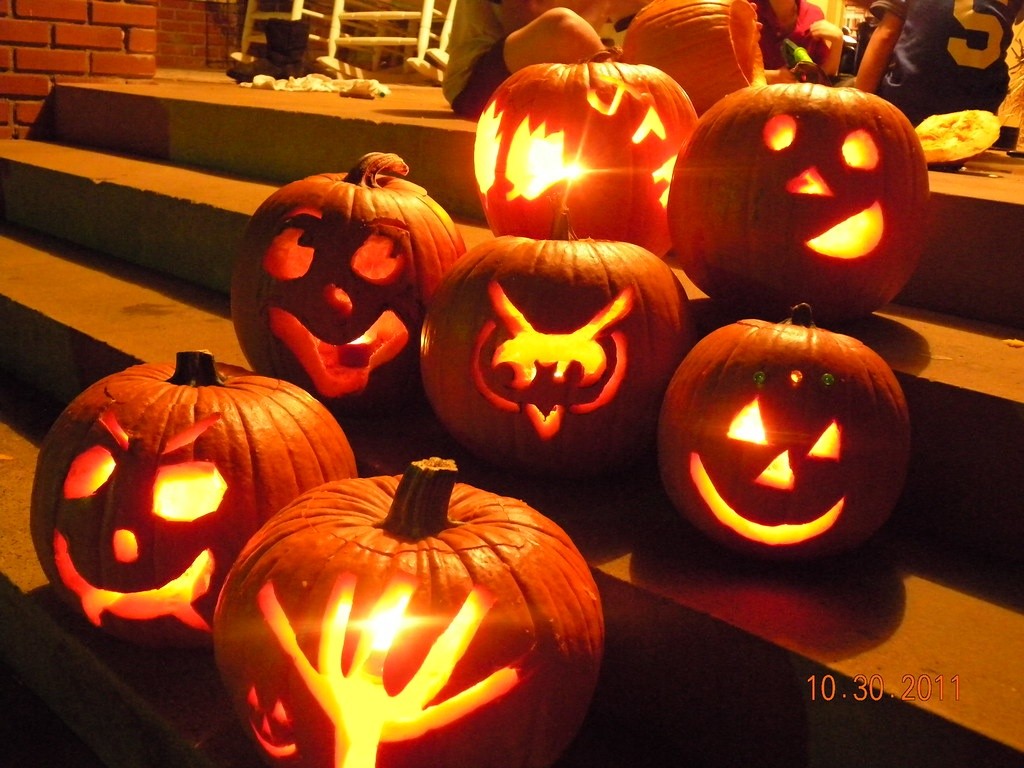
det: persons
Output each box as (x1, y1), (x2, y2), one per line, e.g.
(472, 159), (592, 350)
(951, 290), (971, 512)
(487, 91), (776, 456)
(443, 1), (1023, 161)
